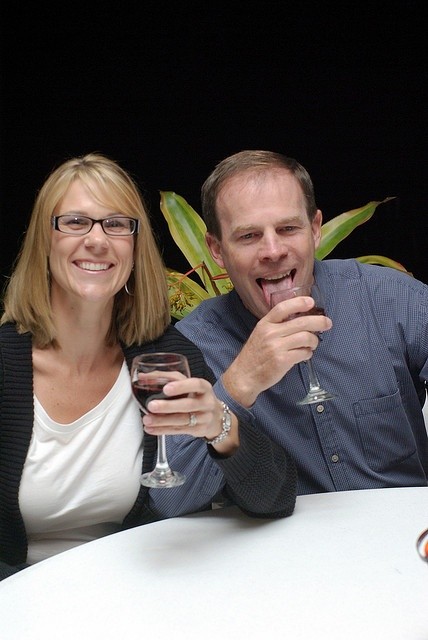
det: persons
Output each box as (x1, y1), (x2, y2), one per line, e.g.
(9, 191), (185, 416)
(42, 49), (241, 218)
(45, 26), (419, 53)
(147, 151), (428, 518)
(0, 153), (297, 589)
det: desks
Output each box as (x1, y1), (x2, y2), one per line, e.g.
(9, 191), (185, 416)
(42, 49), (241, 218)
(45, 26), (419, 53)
(0, 486), (428, 640)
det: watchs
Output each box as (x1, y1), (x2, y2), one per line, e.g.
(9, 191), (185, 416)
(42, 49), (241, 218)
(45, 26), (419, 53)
(207, 401), (232, 448)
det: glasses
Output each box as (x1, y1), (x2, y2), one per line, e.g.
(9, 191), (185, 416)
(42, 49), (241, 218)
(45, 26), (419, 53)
(52, 214), (139, 237)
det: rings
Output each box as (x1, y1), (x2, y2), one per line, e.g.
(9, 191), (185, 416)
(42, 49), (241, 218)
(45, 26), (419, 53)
(188, 413), (198, 427)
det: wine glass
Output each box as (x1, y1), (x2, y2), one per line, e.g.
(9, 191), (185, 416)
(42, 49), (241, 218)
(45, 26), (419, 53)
(270, 283), (340, 407)
(130, 352), (188, 489)
